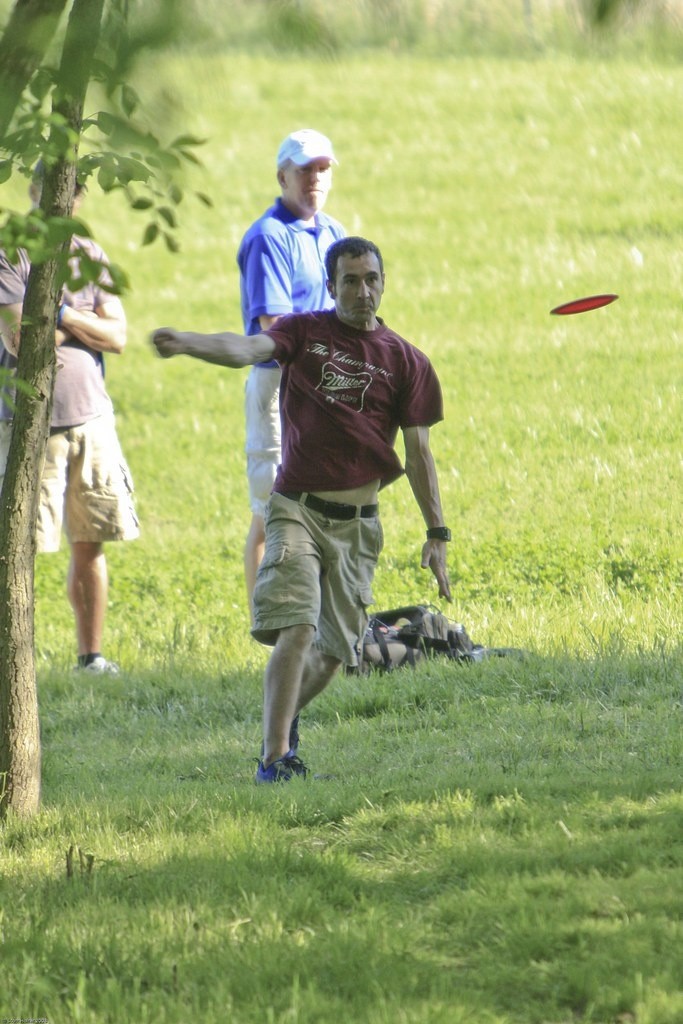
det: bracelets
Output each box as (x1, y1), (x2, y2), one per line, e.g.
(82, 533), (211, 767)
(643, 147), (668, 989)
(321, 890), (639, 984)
(57, 304), (67, 327)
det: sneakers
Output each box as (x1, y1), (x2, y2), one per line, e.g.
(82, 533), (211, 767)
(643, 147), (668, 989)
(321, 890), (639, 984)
(253, 748), (338, 785)
(261, 712), (300, 757)
(77, 658), (124, 680)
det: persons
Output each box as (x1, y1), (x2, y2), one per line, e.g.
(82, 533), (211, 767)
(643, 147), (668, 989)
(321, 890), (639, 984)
(151, 235), (453, 782)
(0, 153), (141, 674)
(235, 127), (350, 636)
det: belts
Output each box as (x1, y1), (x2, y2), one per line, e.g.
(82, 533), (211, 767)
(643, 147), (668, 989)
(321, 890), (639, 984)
(276, 486), (378, 520)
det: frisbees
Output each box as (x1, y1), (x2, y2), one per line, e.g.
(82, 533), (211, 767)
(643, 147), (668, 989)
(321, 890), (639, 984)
(550, 294), (619, 315)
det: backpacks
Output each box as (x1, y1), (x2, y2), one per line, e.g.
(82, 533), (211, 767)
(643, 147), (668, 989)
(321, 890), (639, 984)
(345, 604), (534, 674)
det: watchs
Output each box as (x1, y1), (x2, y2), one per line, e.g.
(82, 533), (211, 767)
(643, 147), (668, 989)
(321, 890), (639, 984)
(427, 527), (451, 541)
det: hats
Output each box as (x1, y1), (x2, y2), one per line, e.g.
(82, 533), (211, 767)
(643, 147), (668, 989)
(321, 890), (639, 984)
(277, 129), (338, 167)
(36, 153), (90, 194)
